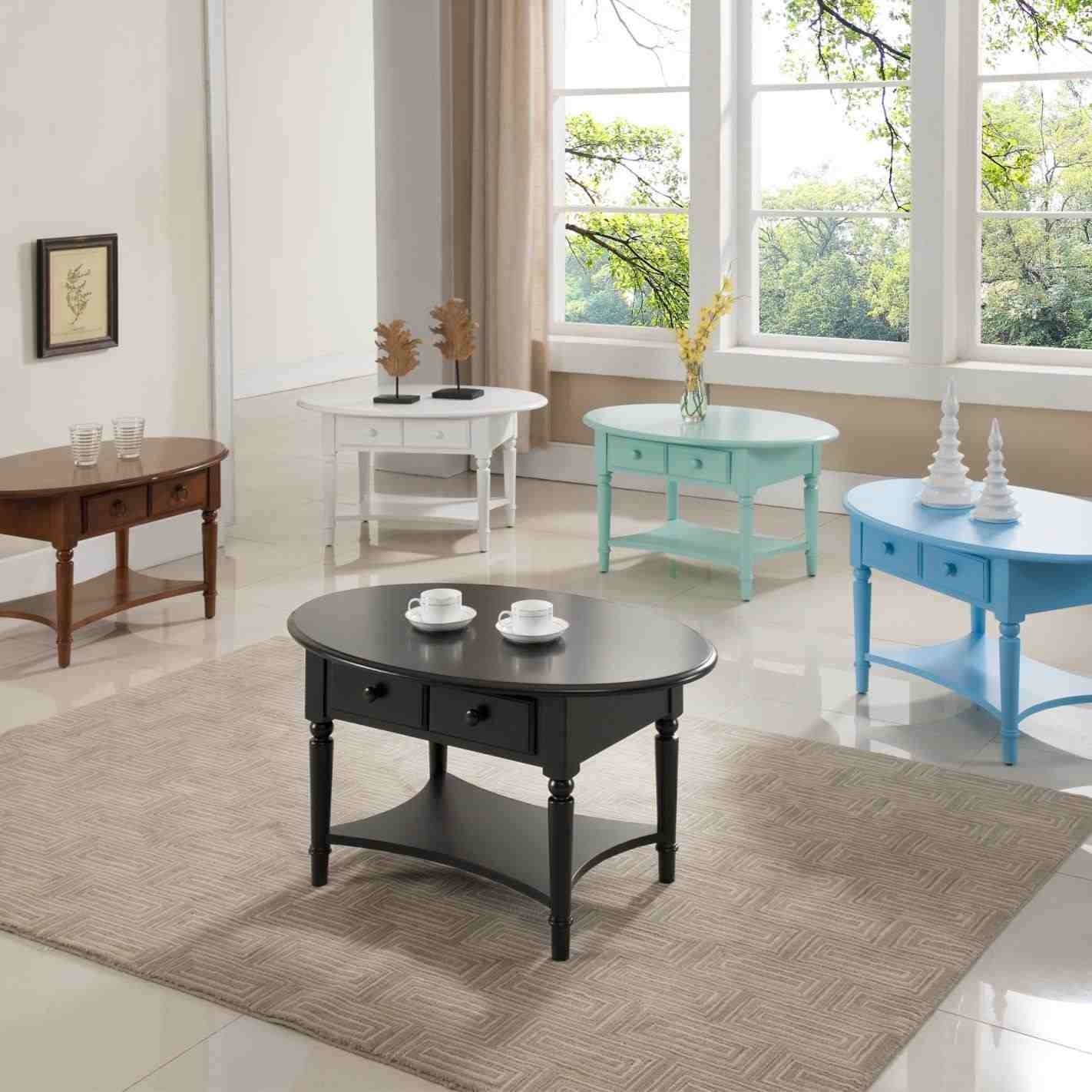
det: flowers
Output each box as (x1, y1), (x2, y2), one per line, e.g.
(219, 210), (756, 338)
(676, 257), (750, 416)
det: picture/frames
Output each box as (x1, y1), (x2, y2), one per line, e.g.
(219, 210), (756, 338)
(36, 233), (119, 358)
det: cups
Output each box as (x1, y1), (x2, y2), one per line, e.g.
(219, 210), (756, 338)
(112, 417), (145, 458)
(70, 423), (103, 467)
(407, 588), (463, 622)
(498, 599), (553, 636)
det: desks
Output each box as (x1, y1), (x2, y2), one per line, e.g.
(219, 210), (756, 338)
(297, 384), (548, 552)
(286, 582), (719, 962)
(843, 477), (1092, 765)
(584, 402), (840, 601)
(0, 436), (229, 668)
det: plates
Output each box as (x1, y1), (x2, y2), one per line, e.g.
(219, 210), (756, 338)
(495, 616), (570, 644)
(405, 606), (477, 631)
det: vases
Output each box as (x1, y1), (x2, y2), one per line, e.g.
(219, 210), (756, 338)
(681, 360), (707, 424)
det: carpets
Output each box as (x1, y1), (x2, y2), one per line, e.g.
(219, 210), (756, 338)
(0, 633), (1092, 1092)
